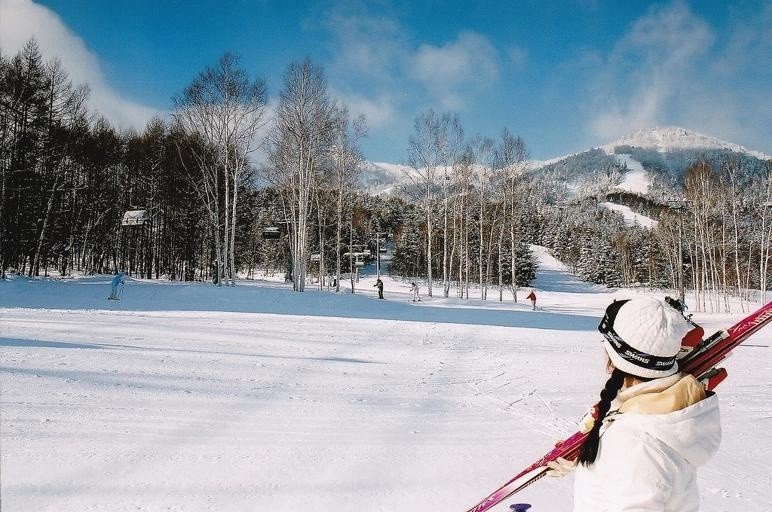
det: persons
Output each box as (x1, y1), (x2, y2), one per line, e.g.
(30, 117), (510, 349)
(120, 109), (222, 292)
(544, 296), (723, 512)
(526, 291), (536, 311)
(410, 283), (420, 301)
(107, 272), (124, 300)
(373, 279), (384, 299)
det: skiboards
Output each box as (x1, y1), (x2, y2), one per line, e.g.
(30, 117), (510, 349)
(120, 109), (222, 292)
(468, 300), (772, 512)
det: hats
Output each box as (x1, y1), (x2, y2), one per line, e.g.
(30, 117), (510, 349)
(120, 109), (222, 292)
(604, 298), (688, 379)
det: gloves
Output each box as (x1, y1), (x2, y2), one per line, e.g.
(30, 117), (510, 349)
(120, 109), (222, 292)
(546, 457), (578, 478)
